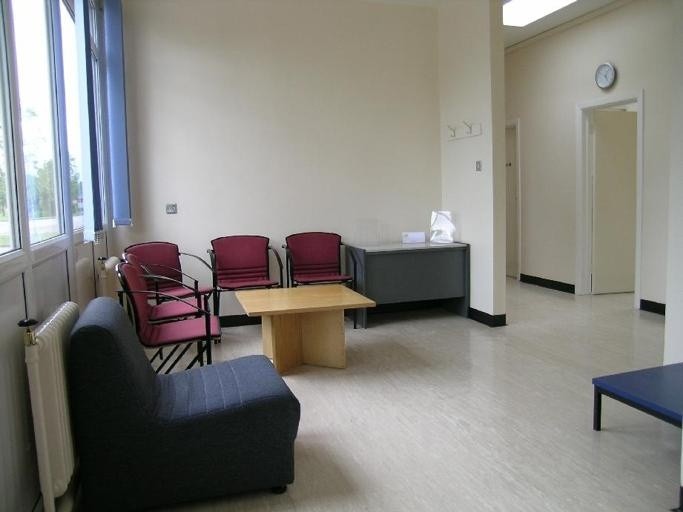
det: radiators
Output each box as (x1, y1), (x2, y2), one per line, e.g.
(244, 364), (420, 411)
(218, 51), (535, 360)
(18, 301), (80, 499)
(97, 256), (127, 314)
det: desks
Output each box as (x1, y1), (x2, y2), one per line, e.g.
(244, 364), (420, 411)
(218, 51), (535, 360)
(341, 235), (471, 328)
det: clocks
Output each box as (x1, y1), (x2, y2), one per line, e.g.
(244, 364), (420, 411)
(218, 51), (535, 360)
(595, 62), (617, 90)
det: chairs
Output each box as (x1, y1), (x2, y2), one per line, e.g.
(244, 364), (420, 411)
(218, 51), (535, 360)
(282, 230), (361, 330)
(121, 241), (221, 345)
(122, 250), (212, 374)
(207, 234), (285, 334)
(116, 261), (223, 377)
(67, 293), (302, 511)
